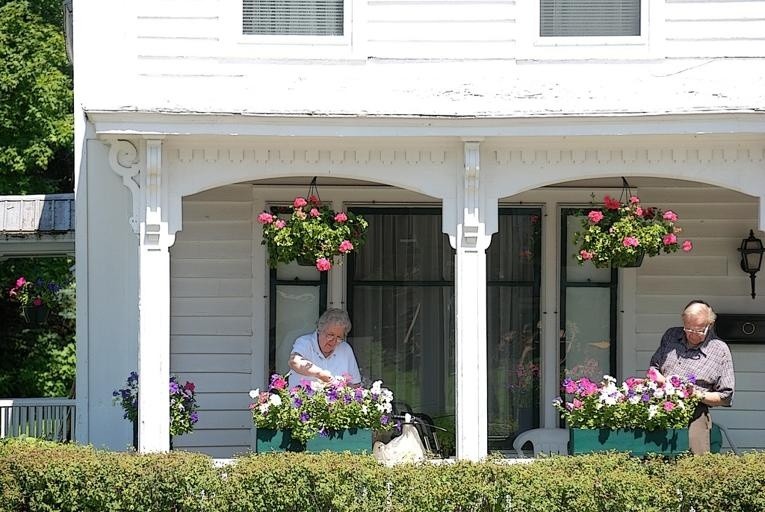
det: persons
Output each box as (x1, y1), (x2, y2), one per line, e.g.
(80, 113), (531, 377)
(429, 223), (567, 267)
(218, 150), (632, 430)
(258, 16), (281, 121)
(646, 301), (735, 455)
(287, 308), (361, 389)
(505, 318), (568, 440)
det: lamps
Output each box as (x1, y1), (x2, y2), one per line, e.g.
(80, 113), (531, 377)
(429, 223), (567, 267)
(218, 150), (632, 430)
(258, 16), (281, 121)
(737, 229), (765, 298)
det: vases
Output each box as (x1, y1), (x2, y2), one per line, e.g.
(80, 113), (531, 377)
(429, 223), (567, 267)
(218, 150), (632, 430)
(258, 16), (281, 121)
(129, 417), (172, 455)
(613, 247), (644, 270)
(294, 250), (322, 267)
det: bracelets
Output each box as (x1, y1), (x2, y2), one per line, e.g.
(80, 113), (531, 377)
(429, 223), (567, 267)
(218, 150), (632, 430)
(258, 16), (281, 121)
(702, 390), (706, 402)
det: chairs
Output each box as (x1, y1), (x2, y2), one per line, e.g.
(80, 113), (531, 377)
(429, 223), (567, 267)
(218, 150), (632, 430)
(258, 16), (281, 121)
(512, 427), (568, 458)
(375, 423), (429, 463)
(522, 318), (579, 363)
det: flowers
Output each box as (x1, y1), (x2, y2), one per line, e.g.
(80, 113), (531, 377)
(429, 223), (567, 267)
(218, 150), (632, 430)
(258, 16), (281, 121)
(6, 272), (62, 311)
(550, 369), (702, 432)
(113, 372), (200, 437)
(516, 213), (542, 269)
(570, 191), (695, 267)
(247, 375), (406, 437)
(257, 194), (370, 270)
(509, 361), (611, 407)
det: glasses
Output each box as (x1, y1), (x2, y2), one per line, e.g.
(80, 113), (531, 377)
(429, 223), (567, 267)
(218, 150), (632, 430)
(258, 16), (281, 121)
(323, 330), (345, 343)
(683, 325), (710, 338)
